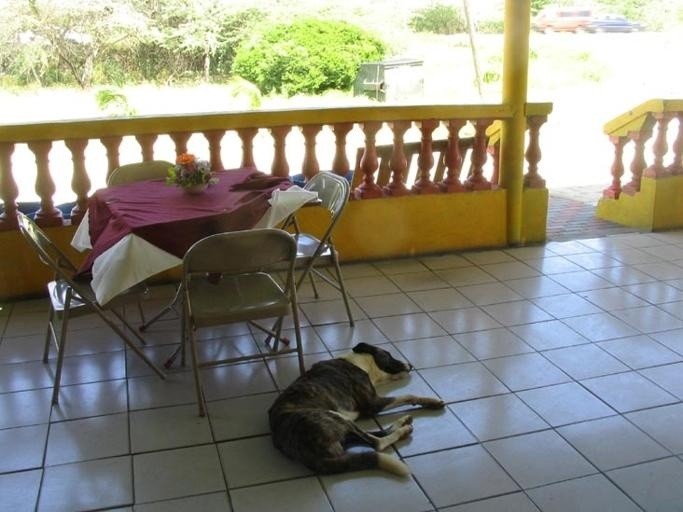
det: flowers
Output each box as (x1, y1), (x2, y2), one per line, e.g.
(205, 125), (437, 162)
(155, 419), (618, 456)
(168, 151), (220, 186)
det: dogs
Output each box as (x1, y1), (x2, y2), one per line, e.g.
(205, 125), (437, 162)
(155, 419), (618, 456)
(267, 342), (446, 478)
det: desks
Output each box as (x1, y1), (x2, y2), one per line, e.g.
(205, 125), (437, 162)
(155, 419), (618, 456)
(90, 166), (322, 369)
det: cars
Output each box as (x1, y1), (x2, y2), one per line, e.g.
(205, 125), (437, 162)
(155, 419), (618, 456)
(585, 14), (647, 33)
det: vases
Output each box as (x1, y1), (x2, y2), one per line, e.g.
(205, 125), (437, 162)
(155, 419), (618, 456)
(187, 183), (208, 193)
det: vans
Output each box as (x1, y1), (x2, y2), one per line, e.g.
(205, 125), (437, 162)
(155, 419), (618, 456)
(532, 5), (594, 35)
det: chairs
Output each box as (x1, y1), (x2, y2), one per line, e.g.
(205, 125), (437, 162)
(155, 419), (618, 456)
(106, 160), (174, 185)
(180, 227), (306, 416)
(15, 210), (167, 403)
(264, 172), (355, 346)
